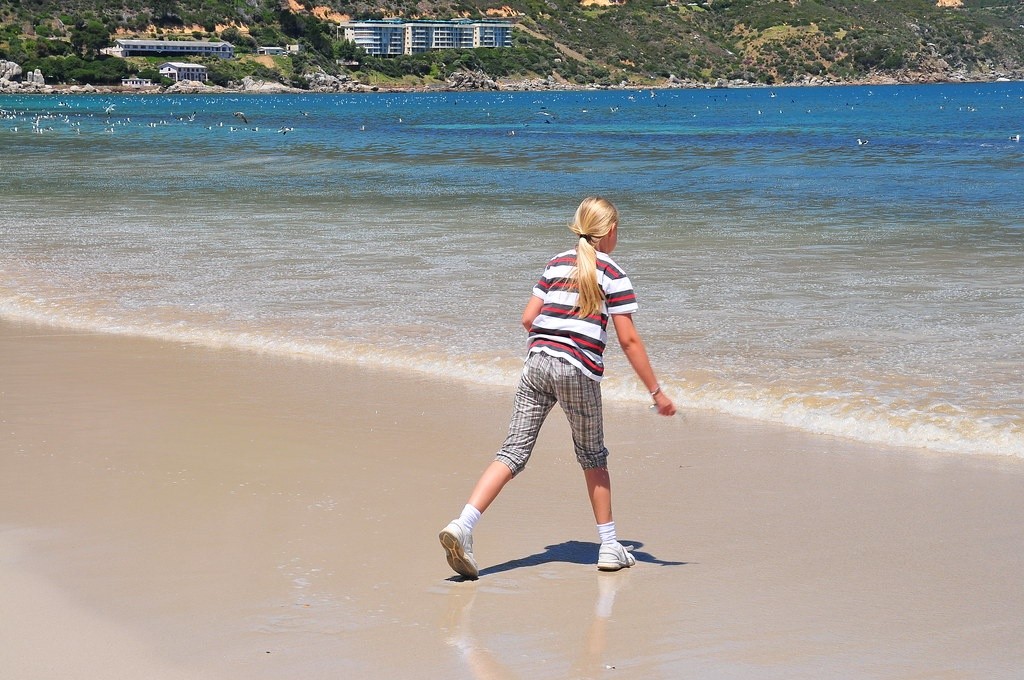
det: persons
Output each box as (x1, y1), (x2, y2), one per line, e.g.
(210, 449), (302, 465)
(438, 196), (674, 579)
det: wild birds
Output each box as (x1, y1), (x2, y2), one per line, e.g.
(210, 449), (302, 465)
(0, 80), (1024, 147)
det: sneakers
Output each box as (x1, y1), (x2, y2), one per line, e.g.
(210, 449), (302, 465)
(438, 519), (480, 580)
(597, 541), (636, 571)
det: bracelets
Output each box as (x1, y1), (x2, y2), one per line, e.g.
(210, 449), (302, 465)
(651, 386), (661, 396)
